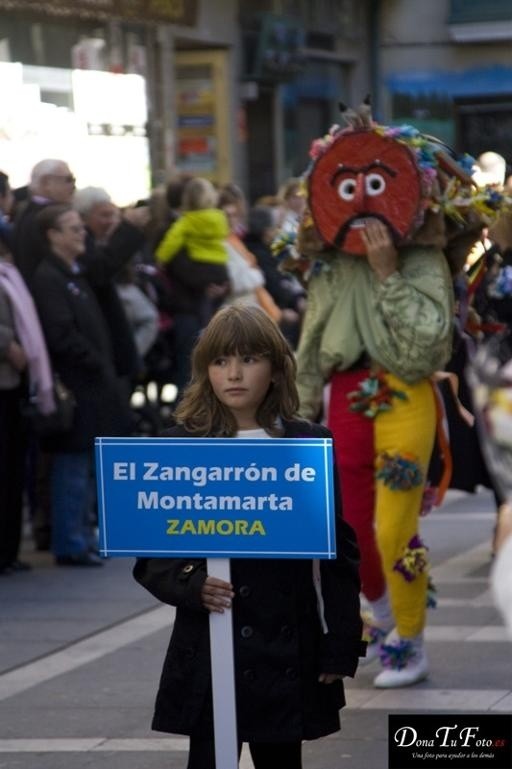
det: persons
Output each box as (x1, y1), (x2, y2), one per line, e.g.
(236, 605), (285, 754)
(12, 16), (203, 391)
(132, 302), (366, 769)
(0, 149), (512, 689)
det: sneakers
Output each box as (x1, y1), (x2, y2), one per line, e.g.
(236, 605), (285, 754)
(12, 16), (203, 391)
(355, 614), (428, 689)
(1, 528), (106, 577)
